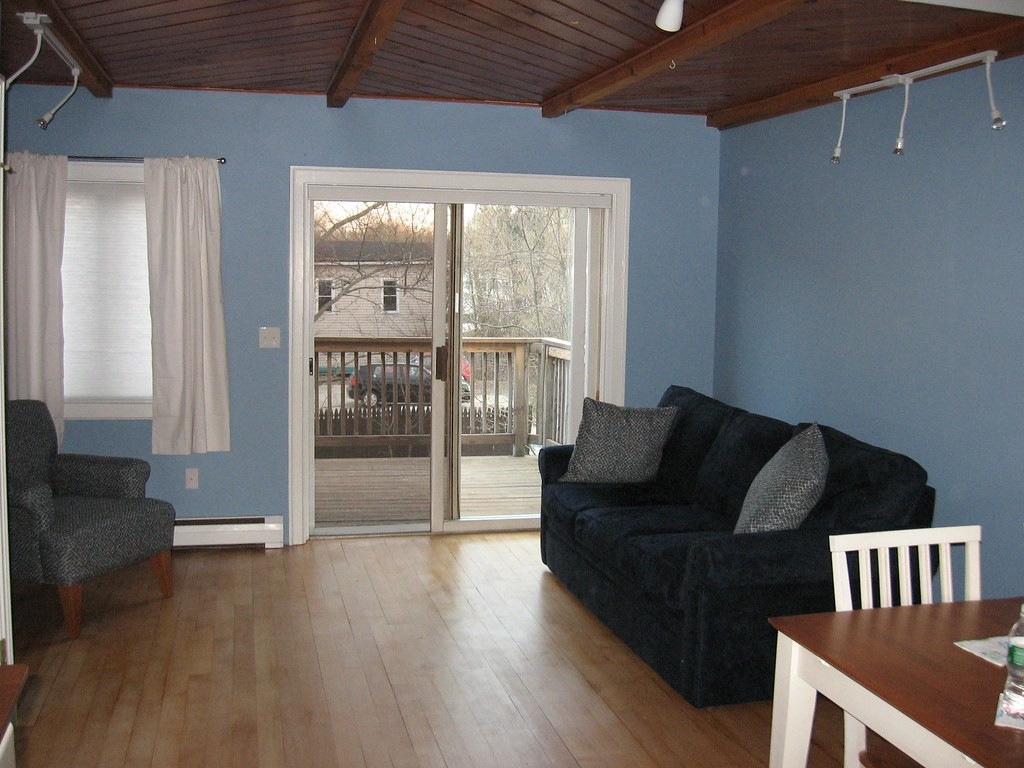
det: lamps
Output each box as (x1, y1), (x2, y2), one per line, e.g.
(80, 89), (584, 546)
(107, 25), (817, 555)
(893, 77), (913, 155)
(36, 69), (80, 131)
(655, 0), (684, 32)
(830, 92), (850, 164)
(981, 55), (1006, 131)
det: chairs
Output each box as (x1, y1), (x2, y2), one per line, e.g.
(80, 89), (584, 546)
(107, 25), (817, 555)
(829, 526), (981, 768)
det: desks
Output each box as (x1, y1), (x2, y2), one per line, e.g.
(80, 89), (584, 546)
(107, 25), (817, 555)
(768, 596), (1024, 768)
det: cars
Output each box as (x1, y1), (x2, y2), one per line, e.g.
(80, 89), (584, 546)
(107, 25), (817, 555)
(346, 352), (472, 408)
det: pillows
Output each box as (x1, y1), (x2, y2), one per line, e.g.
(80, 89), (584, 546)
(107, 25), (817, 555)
(558, 397), (679, 485)
(733, 421), (829, 534)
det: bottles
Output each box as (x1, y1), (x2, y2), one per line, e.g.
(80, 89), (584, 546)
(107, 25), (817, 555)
(1002, 603), (1024, 720)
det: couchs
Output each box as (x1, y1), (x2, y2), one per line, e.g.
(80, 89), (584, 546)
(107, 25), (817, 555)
(538, 384), (941, 712)
(4, 400), (177, 642)
